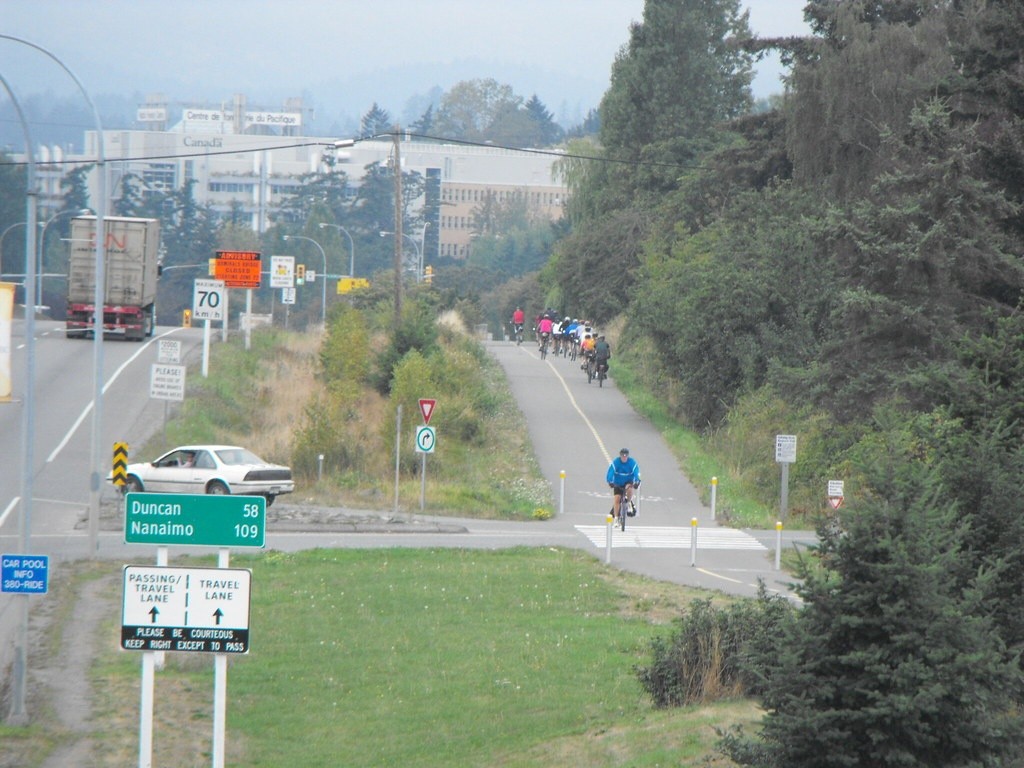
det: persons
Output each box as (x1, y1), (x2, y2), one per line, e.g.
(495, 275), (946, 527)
(537, 313), (552, 352)
(576, 320), (599, 379)
(607, 448), (641, 527)
(177, 453), (197, 469)
(510, 306), (524, 344)
(542, 308), (579, 356)
(595, 337), (611, 387)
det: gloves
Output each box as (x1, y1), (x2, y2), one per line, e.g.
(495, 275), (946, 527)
(610, 483), (614, 488)
(633, 484), (638, 488)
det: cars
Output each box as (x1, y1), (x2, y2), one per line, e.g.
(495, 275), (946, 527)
(110, 448), (294, 508)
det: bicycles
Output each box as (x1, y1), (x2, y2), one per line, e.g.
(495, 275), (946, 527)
(610, 481), (637, 532)
(511, 321), (610, 388)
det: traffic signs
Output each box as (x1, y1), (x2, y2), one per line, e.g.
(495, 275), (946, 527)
(120, 565), (252, 655)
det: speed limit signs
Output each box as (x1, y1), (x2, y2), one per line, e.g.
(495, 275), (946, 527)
(194, 278), (225, 320)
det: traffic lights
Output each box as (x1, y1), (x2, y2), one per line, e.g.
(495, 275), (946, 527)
(297, 264), (305, 285)
(425, 266), (432, 284)
(183, 309), (191, 327)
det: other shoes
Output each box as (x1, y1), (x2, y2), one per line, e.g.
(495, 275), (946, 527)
(626, 501), (633, 514)
(614, 517), (619, 528)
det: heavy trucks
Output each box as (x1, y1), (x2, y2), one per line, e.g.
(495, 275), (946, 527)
(65, 216), (164, 342)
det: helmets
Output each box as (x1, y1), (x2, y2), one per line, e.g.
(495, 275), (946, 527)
(620, 448), (630, 456)
(544, 307), (605, 341)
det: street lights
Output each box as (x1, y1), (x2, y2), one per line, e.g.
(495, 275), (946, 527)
(38, 208), (91, 315)
(0, 222), (47, 246)
(380, 231), (419, 282)
(319, 223), (354, 276)
(283, 235), (326, 322)
(420, 222), (431, 279)
